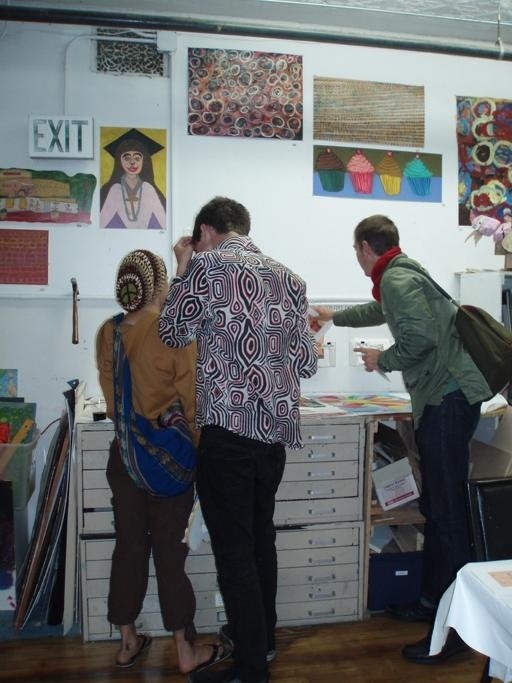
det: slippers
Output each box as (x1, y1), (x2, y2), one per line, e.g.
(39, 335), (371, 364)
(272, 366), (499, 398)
(191, 643), (231, 672)
(115, 634), (152, 668)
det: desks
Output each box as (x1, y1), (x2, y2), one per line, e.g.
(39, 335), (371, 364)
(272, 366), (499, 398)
(458, 557), (512, 683)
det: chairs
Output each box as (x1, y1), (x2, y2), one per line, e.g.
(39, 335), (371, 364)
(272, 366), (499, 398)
(463, 473), (512, 683)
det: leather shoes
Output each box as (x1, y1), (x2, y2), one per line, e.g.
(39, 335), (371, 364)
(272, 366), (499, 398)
(401, 628), (469, 663)
(220, 623), (276, 661)
(188, 670), (270, 683)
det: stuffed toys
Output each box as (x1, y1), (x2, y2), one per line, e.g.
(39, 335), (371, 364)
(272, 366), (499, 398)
(471, 214), (510, 242)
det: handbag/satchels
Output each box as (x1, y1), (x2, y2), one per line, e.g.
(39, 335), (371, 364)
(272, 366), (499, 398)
(455, 305), (511, 394)
(119, 414), (198, 497)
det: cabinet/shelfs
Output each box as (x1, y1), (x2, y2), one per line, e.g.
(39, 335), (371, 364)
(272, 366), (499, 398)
(74, 389), (371, 641)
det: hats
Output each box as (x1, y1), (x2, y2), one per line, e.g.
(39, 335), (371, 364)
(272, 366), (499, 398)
(103, 128), (164, 158)
(116, 248), (165, 313)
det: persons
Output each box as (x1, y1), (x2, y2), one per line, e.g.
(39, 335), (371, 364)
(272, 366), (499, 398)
(99, 139), (167, 229)
(311, 215), (512, 666)
